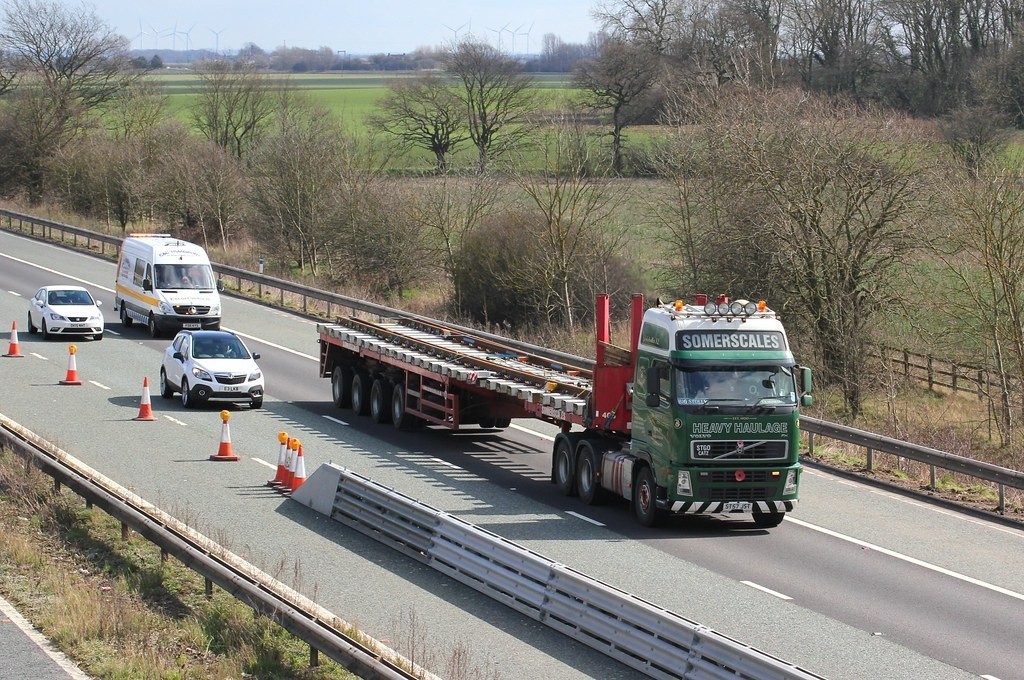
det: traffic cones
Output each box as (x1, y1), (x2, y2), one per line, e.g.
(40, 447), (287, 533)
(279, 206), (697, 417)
(58, 344), (82, 385)
(272, 437), (306, 497)
(1, 320), (25, 357)
(267, 432), (289, 487)
(209, 409), (240, 461)
(131, 376), (159, 422)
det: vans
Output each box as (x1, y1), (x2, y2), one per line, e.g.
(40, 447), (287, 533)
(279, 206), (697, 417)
(113, 233), (225, 339)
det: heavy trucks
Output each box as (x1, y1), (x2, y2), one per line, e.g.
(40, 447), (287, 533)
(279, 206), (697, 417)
(316, 291), (815, 529)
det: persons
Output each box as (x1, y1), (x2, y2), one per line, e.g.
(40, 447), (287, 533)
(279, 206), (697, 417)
(194, 338), (237, 358)
(50, 291), (83, 304)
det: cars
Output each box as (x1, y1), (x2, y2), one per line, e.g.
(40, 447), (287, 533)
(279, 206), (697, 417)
(27, 284), (103, 341)
(159, 329), (265, 409)
